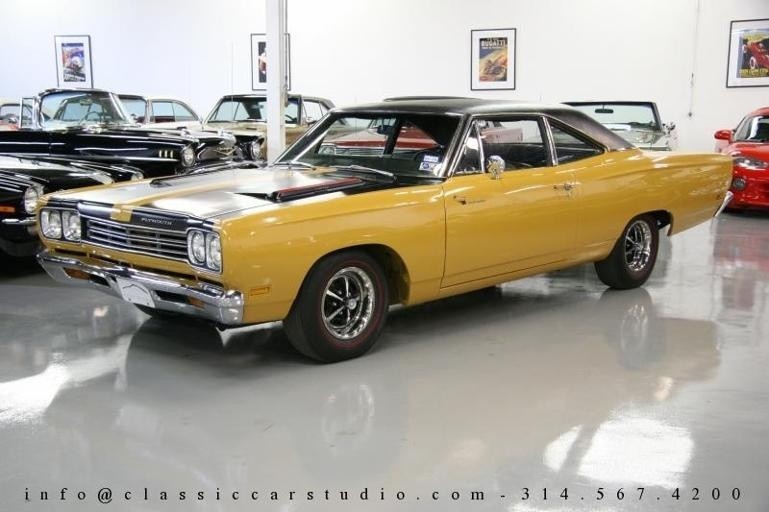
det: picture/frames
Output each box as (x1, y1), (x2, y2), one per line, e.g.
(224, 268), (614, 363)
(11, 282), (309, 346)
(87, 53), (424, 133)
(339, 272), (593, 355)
(470, 27), (517, 91)
(54, 35), (93, 91)
(726, 18), (768, 88)
(249, 32), (291, 92)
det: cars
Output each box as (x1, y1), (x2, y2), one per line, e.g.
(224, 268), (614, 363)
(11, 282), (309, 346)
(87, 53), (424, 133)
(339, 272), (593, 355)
(715, 107), (769, 211)
